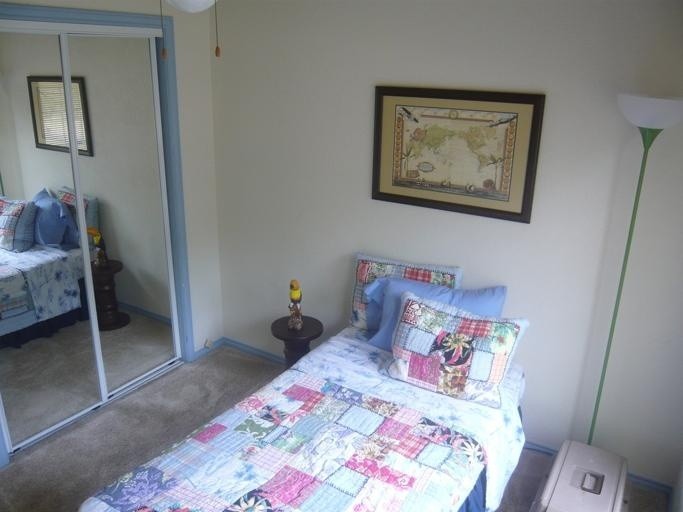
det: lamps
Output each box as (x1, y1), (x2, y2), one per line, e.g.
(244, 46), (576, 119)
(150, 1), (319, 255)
(585, 90), (682, 450)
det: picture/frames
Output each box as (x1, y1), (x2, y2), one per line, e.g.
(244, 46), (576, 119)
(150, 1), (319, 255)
(27, 74), (96, 156)
(369, 84), (547, 226)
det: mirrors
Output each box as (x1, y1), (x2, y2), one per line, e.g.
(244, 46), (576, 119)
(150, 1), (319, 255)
(0, 28), (107, 456)
(64, 28), (179, 405)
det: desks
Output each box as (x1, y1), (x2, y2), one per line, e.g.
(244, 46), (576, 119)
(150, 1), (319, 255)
(269, 316), (324, 370)
(88, 259), (131, 331)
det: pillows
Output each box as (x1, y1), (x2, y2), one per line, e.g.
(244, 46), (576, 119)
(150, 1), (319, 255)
(344, 261), (527, 411)
(1, 186), (104, 254)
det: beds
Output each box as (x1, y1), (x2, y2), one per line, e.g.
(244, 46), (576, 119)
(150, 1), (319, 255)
(1, 247), (107, 339)
(71, 324), (525, 512)
